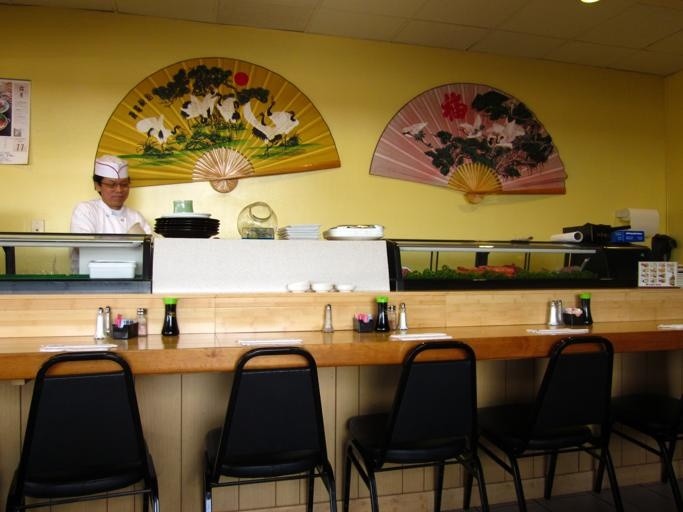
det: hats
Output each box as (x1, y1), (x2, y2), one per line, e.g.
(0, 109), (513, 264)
(95, 155), (129, 179)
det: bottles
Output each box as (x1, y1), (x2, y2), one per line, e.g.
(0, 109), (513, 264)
(578, 294), (592, 324)
(547, 299), (564, 325)
(96, 305), (112, 339)
(398, 301), (407, 330)
(135, 307), (148, 335)
(323, 304), (333, 332)
(163, 298), (180, 336)
(377, 296), (388, 331)
(386, 305), (398, 329)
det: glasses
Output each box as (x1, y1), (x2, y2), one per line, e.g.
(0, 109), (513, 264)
(95, 180), (129, 189)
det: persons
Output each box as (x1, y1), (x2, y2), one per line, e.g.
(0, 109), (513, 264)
(69, 154), (154, 278)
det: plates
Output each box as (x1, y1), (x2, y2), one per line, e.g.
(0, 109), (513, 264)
(287, 282), (356, 292)
(153, 218), (219, 239)
(279, 225), (320, 239)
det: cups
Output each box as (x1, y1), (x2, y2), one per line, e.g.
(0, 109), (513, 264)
(174, 200), (192, 212)
